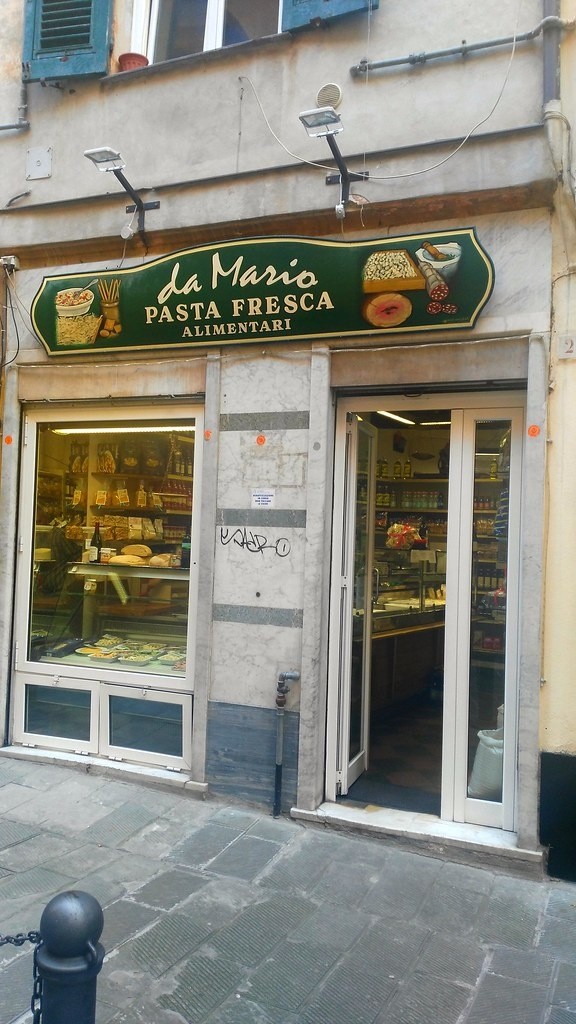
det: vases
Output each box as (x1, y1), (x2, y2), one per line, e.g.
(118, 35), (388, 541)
(118, 53), (150, 72)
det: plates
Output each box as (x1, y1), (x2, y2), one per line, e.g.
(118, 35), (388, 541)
(75, 634), (186, 666)
(88, 522), (102, 563)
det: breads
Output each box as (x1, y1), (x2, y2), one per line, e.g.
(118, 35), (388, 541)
(108, 544), (173, 567)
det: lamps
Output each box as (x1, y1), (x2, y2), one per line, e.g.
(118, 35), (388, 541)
(299, 106), (370, 186)
(83, 146), (161, 212)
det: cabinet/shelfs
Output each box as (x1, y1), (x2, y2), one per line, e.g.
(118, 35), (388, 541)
(31, 433), (511, 711)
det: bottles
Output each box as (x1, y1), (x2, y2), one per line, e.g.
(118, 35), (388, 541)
(474, 496), (494, 510)
(181, 531), (191, 568)
(491, 569), (498, 591)
(477, 569), (483, 590)
(381, 459), (389, 479)
(172, 449), (192, 477)
(401, 491), (443, 509)
(357, 485), (397, 508)
(393, 459), (401, 479)
(404, 460), (411, 478)
(484, 569), (491, 591)
(489, 459), (498, 479)
(498, 569), (505, 589)
(376, 460), (380, 477)
(161, 483), (192, 511)
